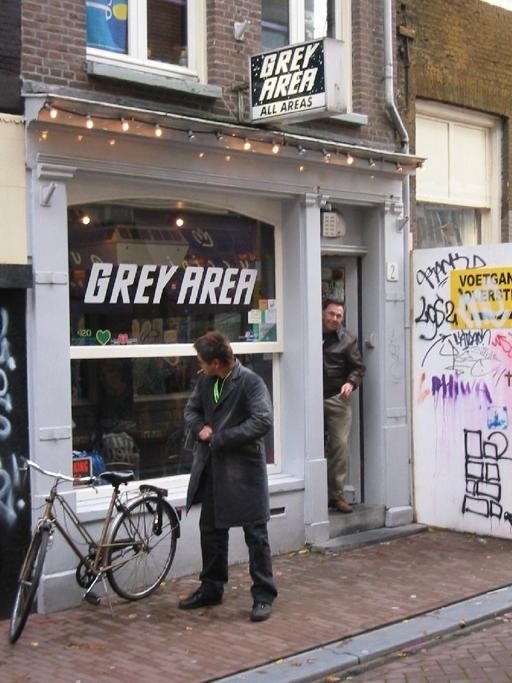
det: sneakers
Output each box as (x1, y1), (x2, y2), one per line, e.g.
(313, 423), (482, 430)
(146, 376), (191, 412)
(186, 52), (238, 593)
(335, 499), (353, 513)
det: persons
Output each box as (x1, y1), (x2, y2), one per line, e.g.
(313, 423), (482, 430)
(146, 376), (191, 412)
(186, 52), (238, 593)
(178, 332), (280, 622)
(321, 297), (366, 514)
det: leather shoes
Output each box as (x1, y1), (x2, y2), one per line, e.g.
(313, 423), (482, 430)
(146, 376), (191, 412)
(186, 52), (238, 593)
(177, 587), (223, 610)
(249, 602), (274, 621)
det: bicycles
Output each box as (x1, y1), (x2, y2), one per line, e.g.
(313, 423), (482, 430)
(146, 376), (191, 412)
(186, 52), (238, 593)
(7, 455), (183, 646)
